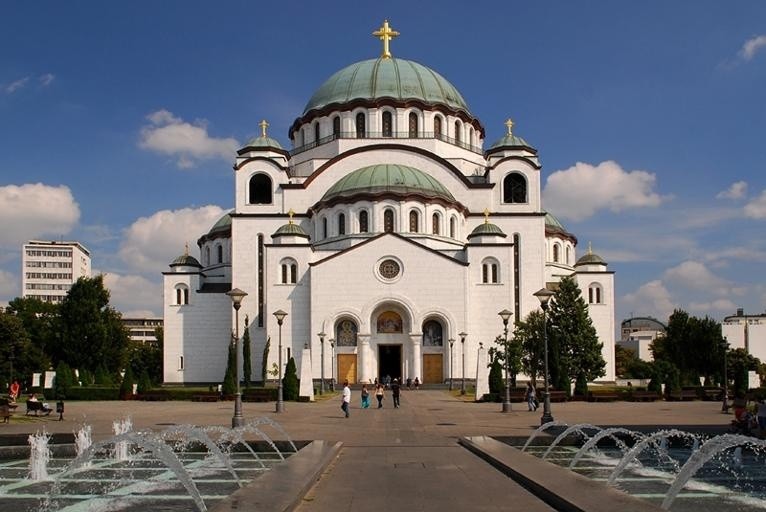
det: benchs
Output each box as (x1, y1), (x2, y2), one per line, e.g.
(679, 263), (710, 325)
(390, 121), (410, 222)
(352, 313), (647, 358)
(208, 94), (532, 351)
(2, 395), (18, 412)
(25, 400), (53, 417)
(0, 400), (13, 424)
(143, 394), (171, 401)
(247, 394), (272, 402)
(499, 388), (736, 402)
(192, 395), (218, 402)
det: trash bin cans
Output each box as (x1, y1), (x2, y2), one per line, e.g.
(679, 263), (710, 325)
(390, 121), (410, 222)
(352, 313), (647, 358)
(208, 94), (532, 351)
(57, 400), (64, 421)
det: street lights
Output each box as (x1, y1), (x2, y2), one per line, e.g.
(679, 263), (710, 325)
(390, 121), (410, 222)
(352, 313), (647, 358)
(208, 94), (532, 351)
(459, 331), (468, 395)
(720, 338), (729, 412)
(273, 309), (288, 412)
(498, 309), (513, 413)
(533, 288), (556, 432)
(226, 287), (248, 428)
(329, 338), (337, 392)
(448, 337), (455, 390)
(317, 332), (326, 395)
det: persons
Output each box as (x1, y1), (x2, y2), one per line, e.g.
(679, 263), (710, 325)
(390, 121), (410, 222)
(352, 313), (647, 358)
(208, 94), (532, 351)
(29, 394), (49, 407)
(753, 397), (766, 435)
(360, 375), (421, 409)
(341, 381), (352, 418)
(524, 382), (537, 411)
(735, 400), (749, 434)
(9, 379), (20, 403)
